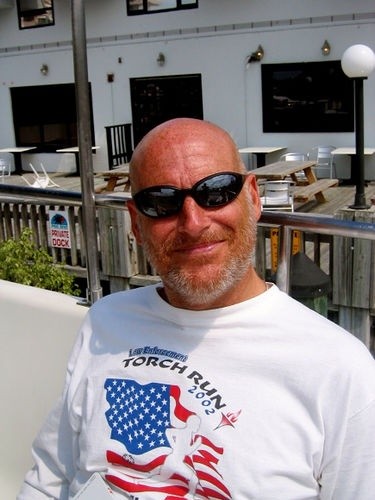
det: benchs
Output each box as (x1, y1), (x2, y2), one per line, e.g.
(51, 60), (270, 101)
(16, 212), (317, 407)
(292, 178), (338, 203)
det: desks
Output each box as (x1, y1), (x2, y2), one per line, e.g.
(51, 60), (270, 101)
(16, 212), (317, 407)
(237, 147), (286, 185)
(332, 148), (375, 186)
(249, 160), (319, 211)
(0, 145), (37, 176)
(56, 146), (100, 177)
(103, 163), (131, 195)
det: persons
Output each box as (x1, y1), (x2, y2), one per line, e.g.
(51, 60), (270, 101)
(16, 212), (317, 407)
(13, 118), (375, 499)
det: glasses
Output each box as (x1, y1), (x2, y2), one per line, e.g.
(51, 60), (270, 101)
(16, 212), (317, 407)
(132, 171), (248, 220)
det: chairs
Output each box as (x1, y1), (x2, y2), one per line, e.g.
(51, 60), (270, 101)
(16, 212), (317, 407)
(311, 148), (336, 180)
(19, 163), (60, 189)
(279, 152), (310, 180)
(256, 180), (294, 212)
(1, 158), (11, 178)
